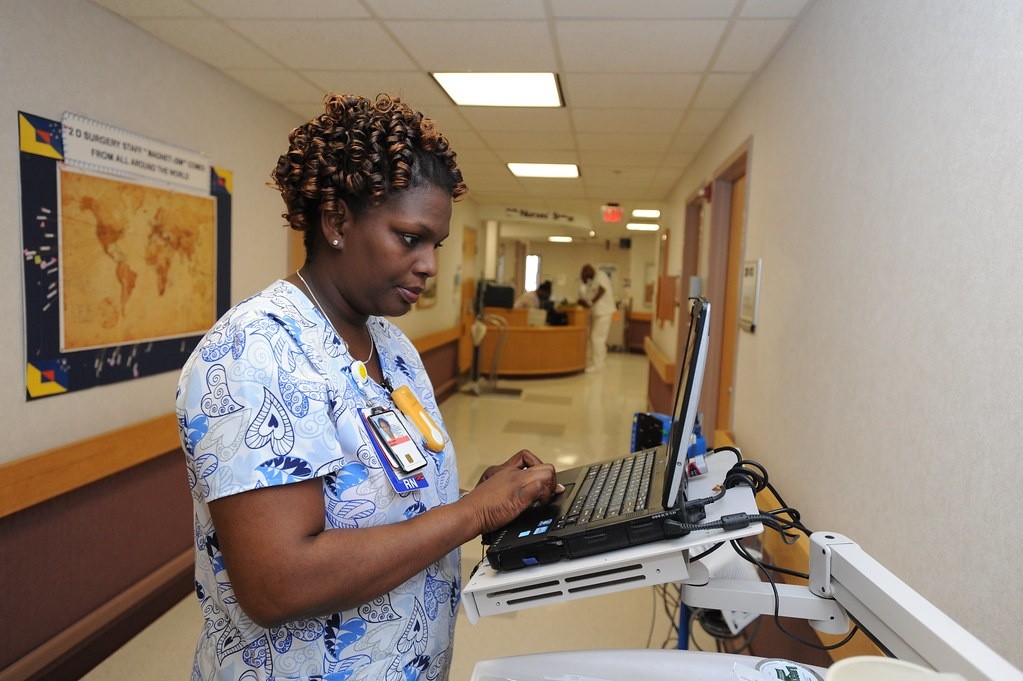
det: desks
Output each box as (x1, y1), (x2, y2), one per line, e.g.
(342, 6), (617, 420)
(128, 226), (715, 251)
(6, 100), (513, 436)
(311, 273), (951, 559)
(460, 445), (1023, 681)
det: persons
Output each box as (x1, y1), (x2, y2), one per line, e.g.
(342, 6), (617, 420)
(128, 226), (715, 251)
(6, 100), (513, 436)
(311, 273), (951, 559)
(512, 280), (553, 309)
(581, 263), (617, 373)
(175, 91), (566, 681)
(377, 418), (398, 441)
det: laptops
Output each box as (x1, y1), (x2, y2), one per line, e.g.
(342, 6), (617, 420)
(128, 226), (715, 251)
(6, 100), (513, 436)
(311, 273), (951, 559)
(486, 296), (711, 570)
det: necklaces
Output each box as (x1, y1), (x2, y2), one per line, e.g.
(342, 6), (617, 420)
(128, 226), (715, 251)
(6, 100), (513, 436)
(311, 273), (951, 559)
(295, 269), (374, 363)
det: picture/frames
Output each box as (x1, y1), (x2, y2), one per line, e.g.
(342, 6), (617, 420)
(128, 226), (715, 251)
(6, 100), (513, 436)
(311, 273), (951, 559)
(739, 259), (762, 333)
(416, 249), (437, 309)
